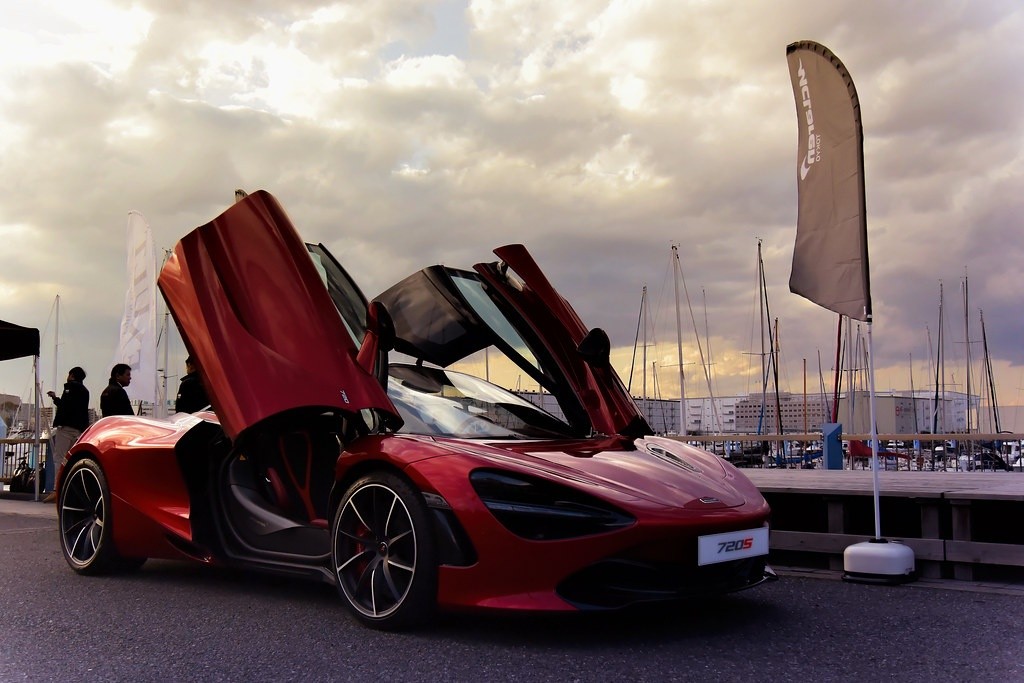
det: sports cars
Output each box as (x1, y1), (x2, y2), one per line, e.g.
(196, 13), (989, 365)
(51, 186), (777, 631)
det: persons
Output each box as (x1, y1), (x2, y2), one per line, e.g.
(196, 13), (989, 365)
(43, 367), (90, 503)
(100, 364), (135, 417)
(176, 356), (208, 414)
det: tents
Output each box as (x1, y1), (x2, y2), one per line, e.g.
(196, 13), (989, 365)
(0, 320), (40, 503)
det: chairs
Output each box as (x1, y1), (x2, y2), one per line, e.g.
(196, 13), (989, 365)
(232, 429), (337, 551)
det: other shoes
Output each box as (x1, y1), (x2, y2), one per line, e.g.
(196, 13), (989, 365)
(43, 491), (56, 503)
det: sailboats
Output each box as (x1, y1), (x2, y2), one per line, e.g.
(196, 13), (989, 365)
(626, 235), (1024, 473)
